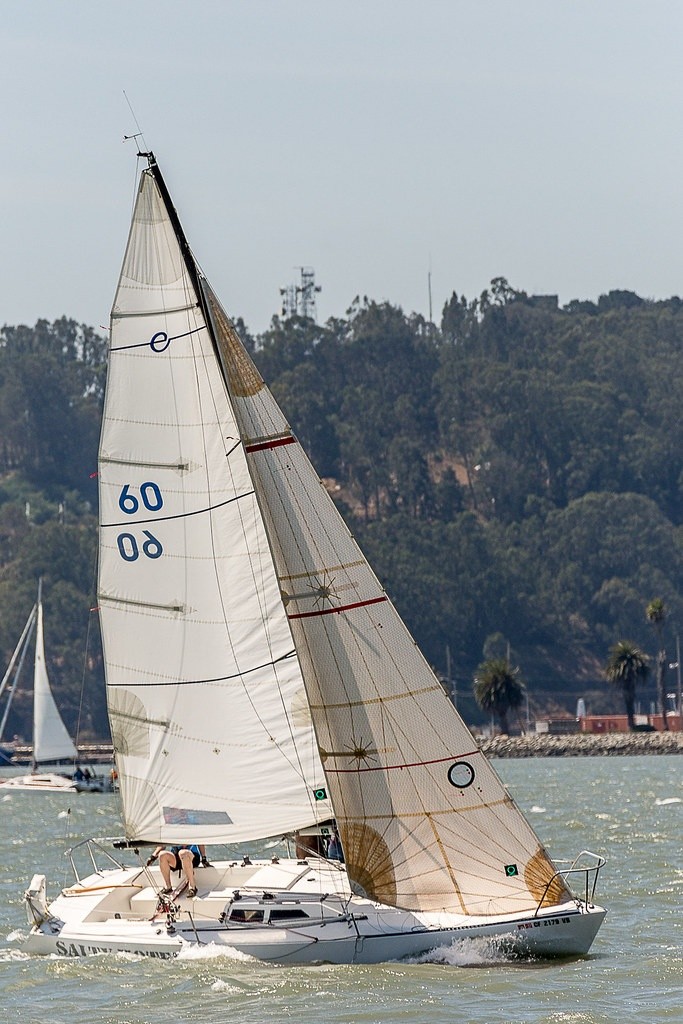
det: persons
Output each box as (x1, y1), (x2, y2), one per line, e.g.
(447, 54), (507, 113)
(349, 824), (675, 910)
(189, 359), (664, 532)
(147, 844), (214, 898)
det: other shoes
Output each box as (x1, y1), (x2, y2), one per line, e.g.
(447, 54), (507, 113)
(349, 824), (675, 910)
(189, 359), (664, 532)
(187, 887), (198, 898)
(157, 886), (173, 896)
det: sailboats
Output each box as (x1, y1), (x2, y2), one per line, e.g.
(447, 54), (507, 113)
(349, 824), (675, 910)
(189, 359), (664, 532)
(2, 137), (610, 971)
(0, 573), (119, 793)
